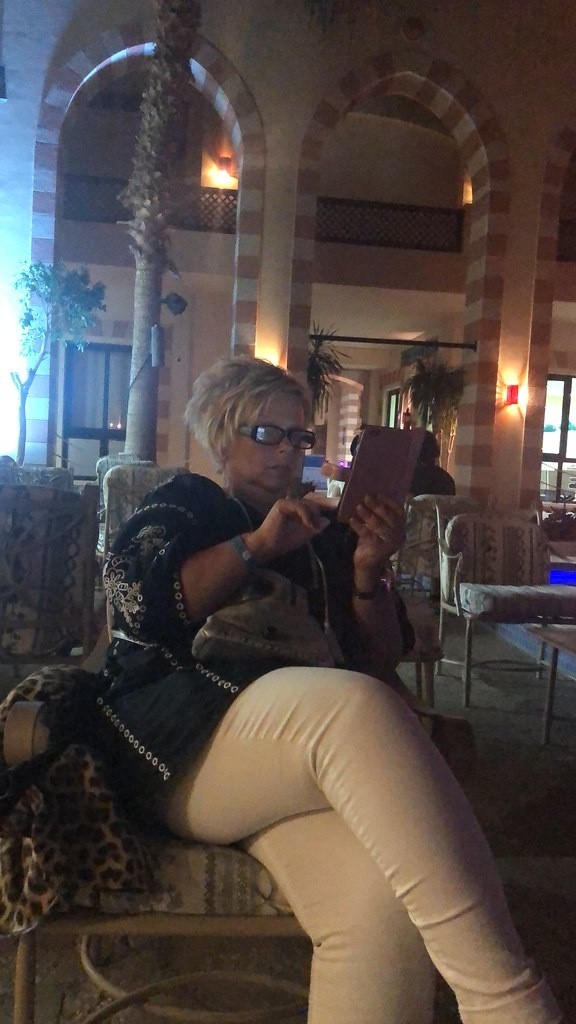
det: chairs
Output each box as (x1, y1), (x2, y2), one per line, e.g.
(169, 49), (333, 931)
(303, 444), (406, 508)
(0, 457), (575, 1023)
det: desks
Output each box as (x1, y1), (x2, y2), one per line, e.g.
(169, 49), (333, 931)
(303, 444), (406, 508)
(524, 623), (576, 746)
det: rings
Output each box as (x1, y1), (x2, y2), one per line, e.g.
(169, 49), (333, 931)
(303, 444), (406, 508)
(376, 526), (393, 542)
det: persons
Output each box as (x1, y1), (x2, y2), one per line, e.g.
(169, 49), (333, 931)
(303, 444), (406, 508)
(350, 424), (366, 455)
(410, 430), (456, 495)
(98, 357), (566, 1024)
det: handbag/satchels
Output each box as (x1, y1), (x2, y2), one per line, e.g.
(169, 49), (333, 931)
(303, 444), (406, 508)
(190, 564), (344, 668)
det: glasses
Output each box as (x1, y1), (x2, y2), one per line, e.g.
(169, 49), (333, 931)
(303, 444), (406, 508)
(238, 424), (316, 450)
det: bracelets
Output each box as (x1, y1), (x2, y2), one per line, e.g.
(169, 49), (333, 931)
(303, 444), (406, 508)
(351, 566), (386, 599)
(230, 534), (262, 571)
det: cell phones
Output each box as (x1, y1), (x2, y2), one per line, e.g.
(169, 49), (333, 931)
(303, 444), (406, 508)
(335, 425), (424, 526)
